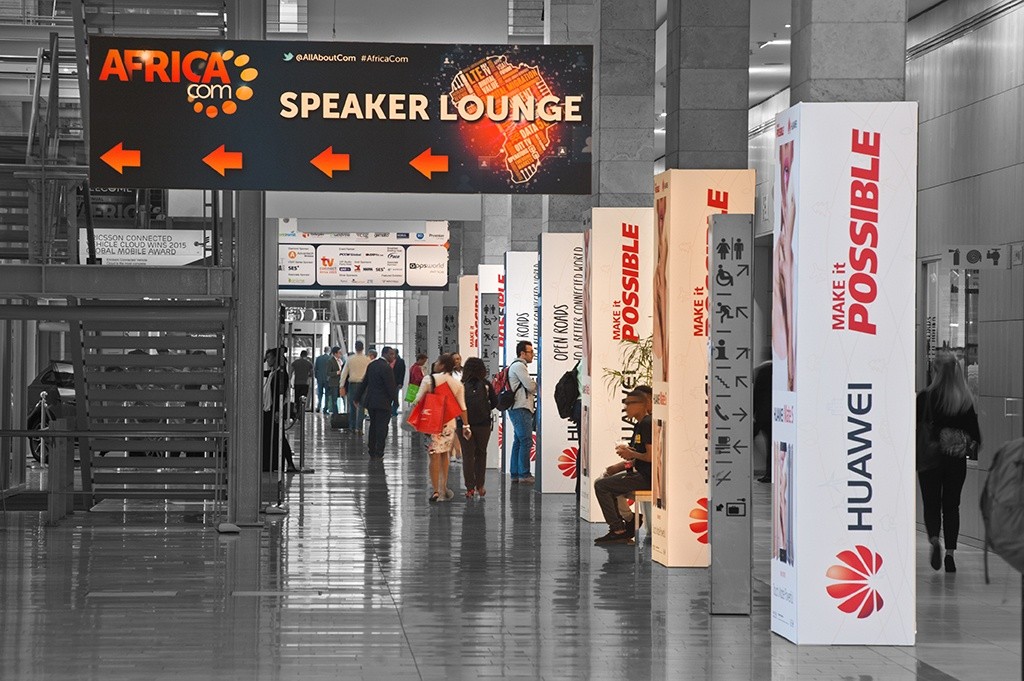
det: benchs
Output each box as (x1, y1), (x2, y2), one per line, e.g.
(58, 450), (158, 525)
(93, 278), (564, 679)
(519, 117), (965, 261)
(623, 491), (651, 543)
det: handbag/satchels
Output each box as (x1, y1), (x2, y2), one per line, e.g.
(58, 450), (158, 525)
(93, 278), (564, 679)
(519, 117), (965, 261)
(406, 374), (464, 434)
(405, 384), (420, 402)
(939, 428), (968, 458)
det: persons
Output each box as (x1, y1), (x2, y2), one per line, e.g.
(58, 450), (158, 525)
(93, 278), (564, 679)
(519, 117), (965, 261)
(595, 385), (652, 547)
(410, 352), (496, 502)
(289, 350), (313, 422)
(315, 341), (406, 460)
(262, 348), (295, 471)
(575, 358), (584, 503)
(917, 349), (981, 573)
(751, 348), (772, 485)
(508, 340), (537, 485)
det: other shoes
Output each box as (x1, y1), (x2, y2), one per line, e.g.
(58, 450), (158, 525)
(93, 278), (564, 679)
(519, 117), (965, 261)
(478, 486), (485, 496)
(931, 543), (942, 570)
(443, 490), (454, 501)
(465, 487), (474, 497)
(429, 493), (440, 502)
(944, 554), (956, 572)
(368, 449), (383, 458)
(594, 530), (629, 544)
(623, 513), (644, 538)
(511, 475), (536, 485)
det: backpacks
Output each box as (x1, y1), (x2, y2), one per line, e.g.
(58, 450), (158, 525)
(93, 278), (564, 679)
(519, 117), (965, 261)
(554, 360), (580, 419)
(492, 360), (527, 410)
(460, 376), (491, 423)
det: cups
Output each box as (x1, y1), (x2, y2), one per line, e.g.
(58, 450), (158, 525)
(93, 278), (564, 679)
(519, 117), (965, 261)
(616, 439), (630, 448)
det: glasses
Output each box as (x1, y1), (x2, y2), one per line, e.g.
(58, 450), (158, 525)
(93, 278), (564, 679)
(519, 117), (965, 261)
(625, 401), (644, 405)
